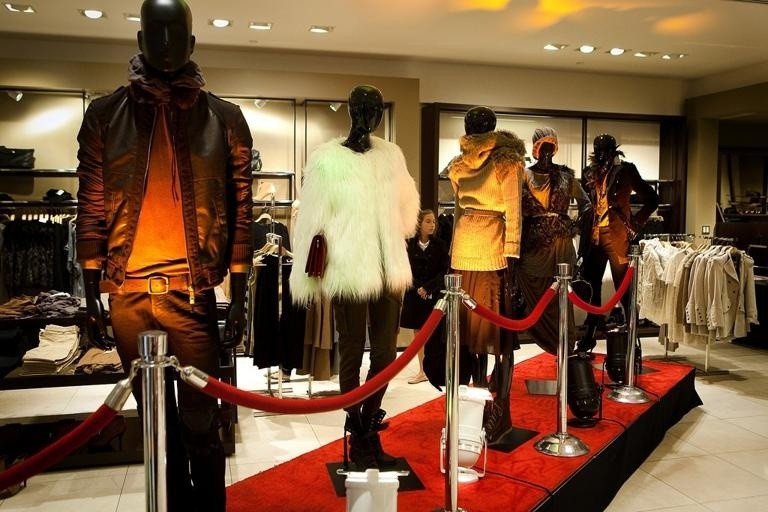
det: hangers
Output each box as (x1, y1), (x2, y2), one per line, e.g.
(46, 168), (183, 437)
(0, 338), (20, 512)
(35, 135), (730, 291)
(253, 199), (279, 224)
(5, 208), (77, 224)
(253, 233), (293, 267)
(645, 234), (698, 252)
(696, 236), (741, 257)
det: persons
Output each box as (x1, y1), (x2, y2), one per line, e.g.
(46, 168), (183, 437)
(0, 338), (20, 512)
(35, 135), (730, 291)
(448, 105), (525, 447)
(576, 134), (660, 374)
(75, 0), (254, 511)
(514, 126), (593, 360)
(399, 209), (450, 384)
(288, 84), (422, 473)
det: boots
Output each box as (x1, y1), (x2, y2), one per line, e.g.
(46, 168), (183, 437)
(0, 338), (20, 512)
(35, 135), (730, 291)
(482, 393), (515, 447)
(344, 408), (397, 472)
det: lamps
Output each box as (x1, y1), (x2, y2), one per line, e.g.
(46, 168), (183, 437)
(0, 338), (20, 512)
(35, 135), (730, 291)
(605, 327), (642, 390)
(335, 468), (410, 512)
(556, 353), (604, 428)
(439, 384), (494, 484)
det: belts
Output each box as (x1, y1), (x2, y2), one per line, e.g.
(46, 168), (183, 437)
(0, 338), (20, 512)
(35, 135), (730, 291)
(98, 272), (225, 295)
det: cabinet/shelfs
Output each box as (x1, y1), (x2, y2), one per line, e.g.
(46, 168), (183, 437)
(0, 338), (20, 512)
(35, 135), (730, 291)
(0, 169), (295, 209)
(421, 102), (688, 243)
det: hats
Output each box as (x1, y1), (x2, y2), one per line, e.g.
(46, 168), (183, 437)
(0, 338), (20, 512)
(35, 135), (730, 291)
(532, 126), (561, 160)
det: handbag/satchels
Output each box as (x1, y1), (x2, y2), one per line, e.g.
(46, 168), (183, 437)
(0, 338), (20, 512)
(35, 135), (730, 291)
(305, 234), (328, 279)
